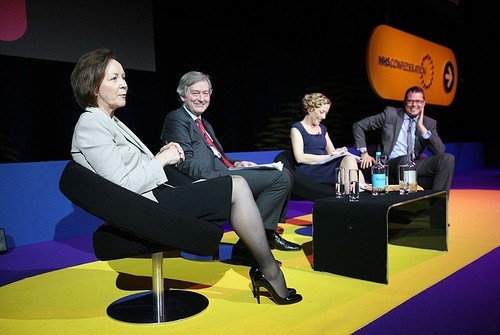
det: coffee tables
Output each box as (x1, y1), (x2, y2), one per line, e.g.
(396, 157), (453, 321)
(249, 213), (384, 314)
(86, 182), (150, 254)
(313, 191), (449, 284)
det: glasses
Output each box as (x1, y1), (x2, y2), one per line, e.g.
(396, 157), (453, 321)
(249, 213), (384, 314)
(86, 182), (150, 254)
(405, 100), (423, 105)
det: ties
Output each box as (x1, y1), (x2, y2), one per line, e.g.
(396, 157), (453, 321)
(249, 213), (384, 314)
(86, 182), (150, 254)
(196, 118), (235, 168)
(407, 118), (414, 161)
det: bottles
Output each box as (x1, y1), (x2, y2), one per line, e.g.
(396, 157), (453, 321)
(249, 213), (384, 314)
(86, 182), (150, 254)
(404, 154), (417, 193)
(371, 152), (386, 195)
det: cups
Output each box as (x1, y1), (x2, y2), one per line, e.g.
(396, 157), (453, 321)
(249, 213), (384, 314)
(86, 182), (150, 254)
(398, 165), (409, 196)
(349, 169), (359, 201)
(335, 167), (345, 198)
(383, 165), (389, 186)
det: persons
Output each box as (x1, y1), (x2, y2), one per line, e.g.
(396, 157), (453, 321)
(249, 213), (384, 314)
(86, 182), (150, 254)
(71, 49), (303, 305)
(352, 86), (455, 227)
(291, 92), (365, 194)
(161, 72), (302, 266)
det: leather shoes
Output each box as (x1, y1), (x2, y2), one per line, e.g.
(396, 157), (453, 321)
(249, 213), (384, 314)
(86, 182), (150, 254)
(266, 230), (301, 251)
(232, 244), (282, 267)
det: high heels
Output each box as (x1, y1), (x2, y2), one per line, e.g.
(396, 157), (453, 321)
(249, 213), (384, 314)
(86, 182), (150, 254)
(253, 270), (302, 305)
(249, 263), (296, 298)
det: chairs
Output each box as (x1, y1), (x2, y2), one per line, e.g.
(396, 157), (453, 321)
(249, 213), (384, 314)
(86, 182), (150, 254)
(275, 151), (335, 224)
(360, 143), (434, 190)
(59, 161), (224, 323)
(151, 138), (221, 260)
